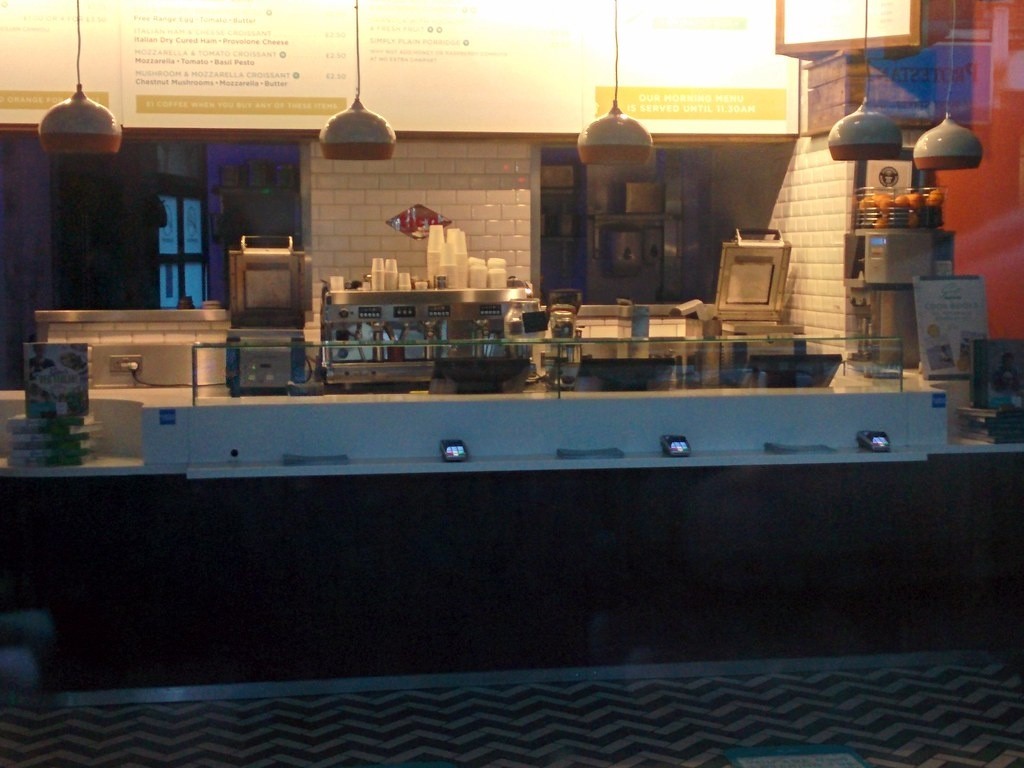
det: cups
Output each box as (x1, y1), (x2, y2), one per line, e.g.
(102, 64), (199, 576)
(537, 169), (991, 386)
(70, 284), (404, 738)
(364, 222), (469, 292)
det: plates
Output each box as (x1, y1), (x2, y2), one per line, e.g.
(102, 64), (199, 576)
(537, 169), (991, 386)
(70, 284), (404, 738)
(469, 254), (508, 289)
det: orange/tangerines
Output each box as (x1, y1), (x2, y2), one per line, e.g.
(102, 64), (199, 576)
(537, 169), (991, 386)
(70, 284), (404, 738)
(859, 190), (944, 228)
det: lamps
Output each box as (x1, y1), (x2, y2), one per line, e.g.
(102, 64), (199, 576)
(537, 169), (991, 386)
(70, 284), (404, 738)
(912, 0), (983, 171)
(827, 0), (902, 160)
(578, 0), (653, 167)
(38, 0), (123, 153)
(319, 0), (397, 161)
